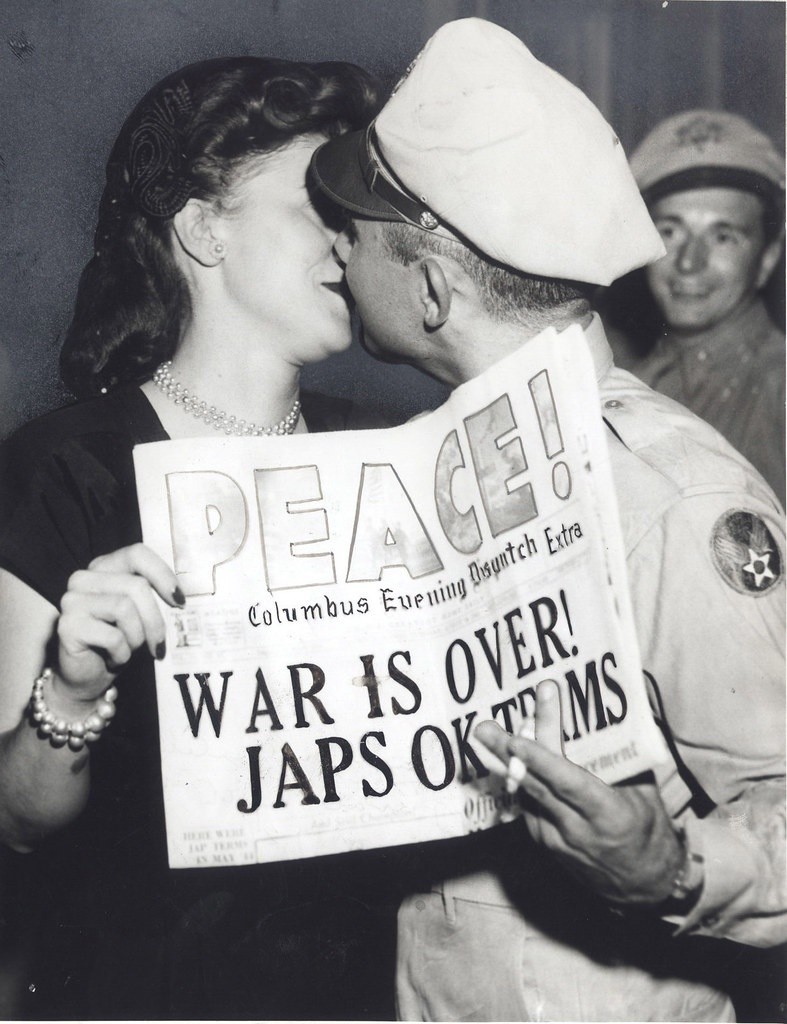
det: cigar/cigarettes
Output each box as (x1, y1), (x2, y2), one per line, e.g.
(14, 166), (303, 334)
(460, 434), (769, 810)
(506, 723), (534, 791)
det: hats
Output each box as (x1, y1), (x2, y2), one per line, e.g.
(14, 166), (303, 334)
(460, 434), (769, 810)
(628, 107), (785, 203)
(311, 18), (668, 285)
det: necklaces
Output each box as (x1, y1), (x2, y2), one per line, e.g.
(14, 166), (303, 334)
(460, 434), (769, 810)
(152, 361), (301, 436)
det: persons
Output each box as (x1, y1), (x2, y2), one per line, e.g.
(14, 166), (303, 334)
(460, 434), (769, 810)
(629, 111), (787, 503)
(0, 57), (397, 1021)
(309, 17), (785, 1021)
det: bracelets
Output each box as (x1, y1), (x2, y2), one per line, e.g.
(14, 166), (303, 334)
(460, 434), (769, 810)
(605, 819), (702, 919)
(32, 669), (117, 746)
